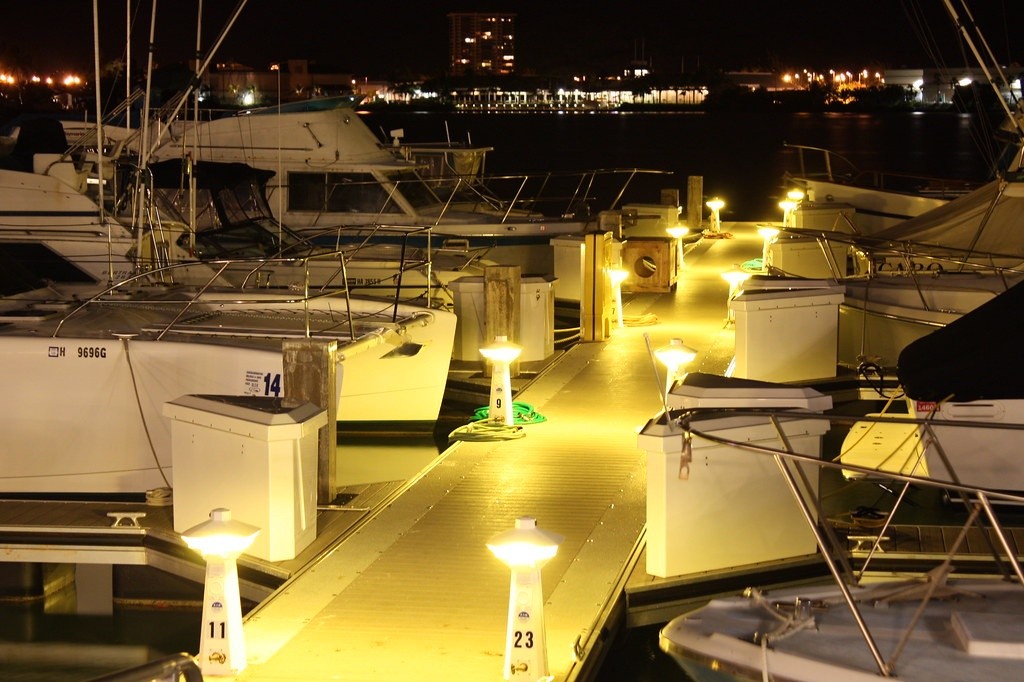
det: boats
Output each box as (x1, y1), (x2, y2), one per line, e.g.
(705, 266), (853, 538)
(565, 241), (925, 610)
(0, 0), (1023, 682)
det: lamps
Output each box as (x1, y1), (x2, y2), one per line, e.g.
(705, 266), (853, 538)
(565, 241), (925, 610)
(478, 335), (523, 427)
(486, 517), (566, 682)
(722, 264), (752, 331)
(181, 509), (261, 675)
(653, 338), (698, 406)
